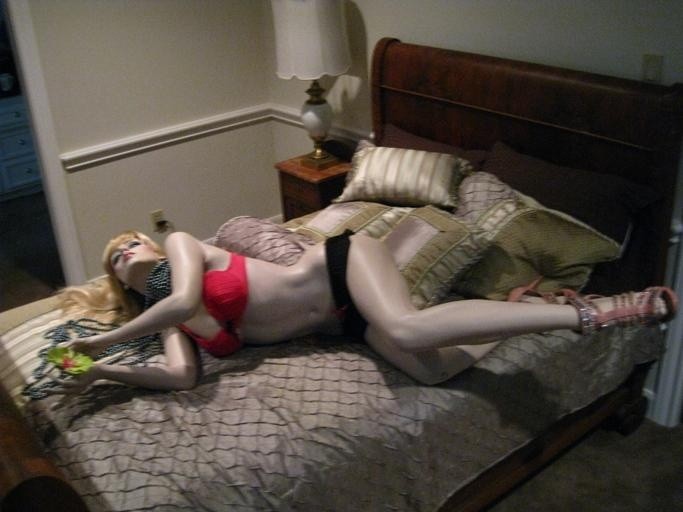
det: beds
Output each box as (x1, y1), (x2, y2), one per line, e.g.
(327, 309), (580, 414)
(0, 36), (683, 512)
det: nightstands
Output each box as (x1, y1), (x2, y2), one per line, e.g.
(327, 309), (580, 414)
(275, 139), (358, 224)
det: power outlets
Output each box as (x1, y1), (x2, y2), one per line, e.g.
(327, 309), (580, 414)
(151, 210), (165, 231)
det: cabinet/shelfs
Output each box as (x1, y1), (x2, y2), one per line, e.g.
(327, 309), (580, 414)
(0, 79), (42, 196)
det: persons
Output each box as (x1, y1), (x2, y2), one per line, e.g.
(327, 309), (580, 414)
(52, 229), (678, 391)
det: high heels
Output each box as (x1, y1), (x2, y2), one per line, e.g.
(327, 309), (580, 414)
(510, 275), (677, 338)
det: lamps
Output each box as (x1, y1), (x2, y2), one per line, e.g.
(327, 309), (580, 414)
(270, 0), (352, 170)
